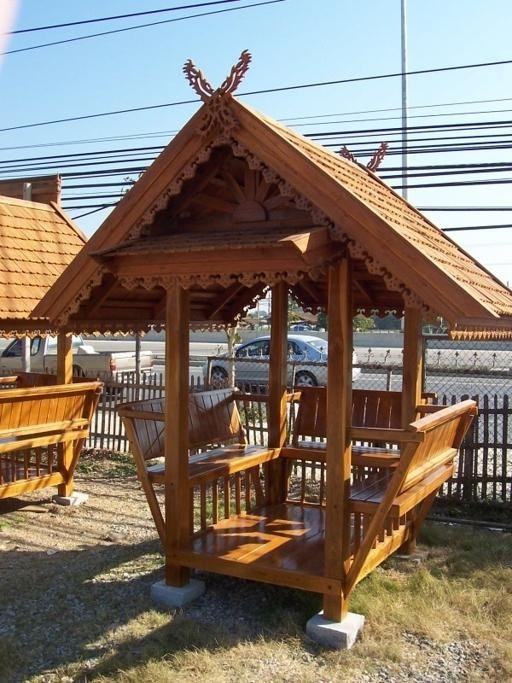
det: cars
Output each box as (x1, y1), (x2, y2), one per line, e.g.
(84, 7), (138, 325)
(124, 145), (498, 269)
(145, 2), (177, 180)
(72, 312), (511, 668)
(203, 333), (362, 389)
(290, 325), (313, 331)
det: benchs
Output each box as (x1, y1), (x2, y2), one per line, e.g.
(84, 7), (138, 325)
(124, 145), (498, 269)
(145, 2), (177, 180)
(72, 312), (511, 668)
(345, 397), (480, 599)
(0, 371), (105, 499)
(117, 386), (279, 544)
(285, 385), (438, 506)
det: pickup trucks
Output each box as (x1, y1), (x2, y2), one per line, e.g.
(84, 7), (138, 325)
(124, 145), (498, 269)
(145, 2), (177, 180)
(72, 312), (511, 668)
(0, 334), (157, 394)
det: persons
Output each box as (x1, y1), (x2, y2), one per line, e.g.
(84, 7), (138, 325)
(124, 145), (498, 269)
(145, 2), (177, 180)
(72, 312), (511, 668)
(287, 343), (297, 355)
